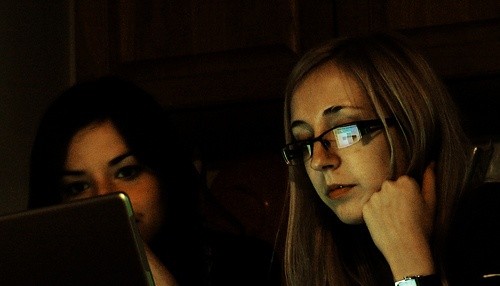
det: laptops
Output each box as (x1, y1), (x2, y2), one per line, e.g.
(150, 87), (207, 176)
(0, 191), (157, 286)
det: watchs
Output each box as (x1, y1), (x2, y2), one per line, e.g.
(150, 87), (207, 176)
(390, 271), (441, 286)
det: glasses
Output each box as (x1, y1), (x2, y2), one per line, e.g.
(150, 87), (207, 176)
(280, 118), (393, 168)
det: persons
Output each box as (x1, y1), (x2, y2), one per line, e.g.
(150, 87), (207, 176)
(278, 33), (500, 286)
(26, 81), (283, 286)
